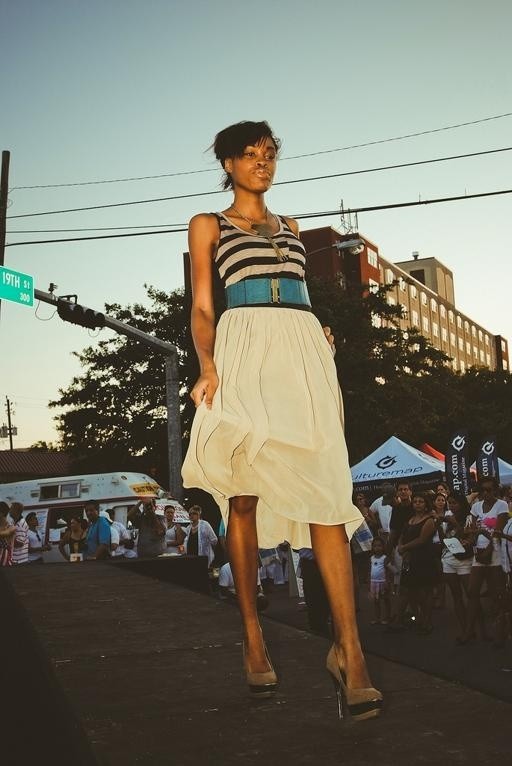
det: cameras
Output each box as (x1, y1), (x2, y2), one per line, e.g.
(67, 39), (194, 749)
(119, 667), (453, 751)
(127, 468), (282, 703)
(442, 516), (449, 522)
(146, 504), (153, 511)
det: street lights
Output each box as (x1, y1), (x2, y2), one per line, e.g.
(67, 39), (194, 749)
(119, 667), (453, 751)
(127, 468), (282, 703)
(307, 231), (369, 263)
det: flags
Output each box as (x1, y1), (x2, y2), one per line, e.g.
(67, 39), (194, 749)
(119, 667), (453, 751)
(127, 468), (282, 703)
(445, 433), (469, 497)
(475, 434), (499, 486)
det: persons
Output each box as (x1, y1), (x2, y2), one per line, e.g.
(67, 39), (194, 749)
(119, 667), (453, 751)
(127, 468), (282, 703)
(180, 120), (383, 719)
(0, 479), (511, 649)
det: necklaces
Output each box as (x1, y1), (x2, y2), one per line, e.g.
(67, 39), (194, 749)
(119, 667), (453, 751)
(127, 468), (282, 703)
(230, 204), (288, 260)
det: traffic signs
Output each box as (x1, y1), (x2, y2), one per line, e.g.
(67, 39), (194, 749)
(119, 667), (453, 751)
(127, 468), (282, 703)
(0, 265), (35, 308)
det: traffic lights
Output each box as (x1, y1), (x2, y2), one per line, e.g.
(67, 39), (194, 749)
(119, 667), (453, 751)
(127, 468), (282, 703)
(59, 299), (106, 330)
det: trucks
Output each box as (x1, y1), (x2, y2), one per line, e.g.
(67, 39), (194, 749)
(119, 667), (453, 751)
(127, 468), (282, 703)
(0, 470), (192, 562)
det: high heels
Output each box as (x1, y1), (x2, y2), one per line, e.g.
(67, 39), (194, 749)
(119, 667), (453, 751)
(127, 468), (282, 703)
(327, 642), (384, 722)
(243, 638), (279, 699)
